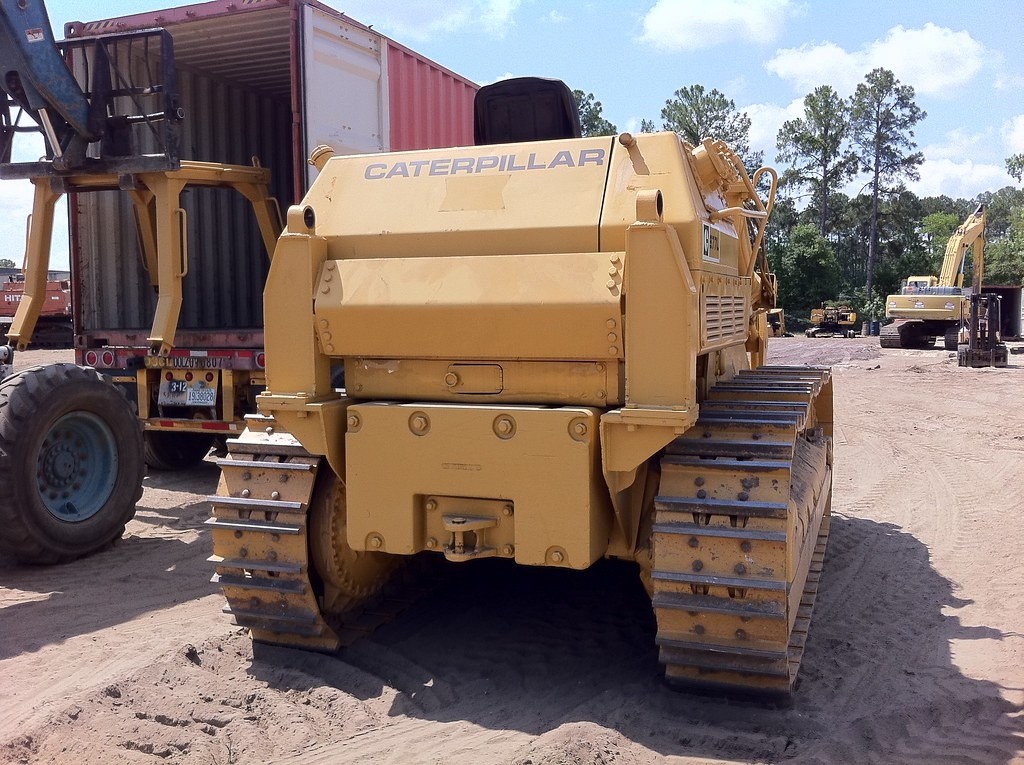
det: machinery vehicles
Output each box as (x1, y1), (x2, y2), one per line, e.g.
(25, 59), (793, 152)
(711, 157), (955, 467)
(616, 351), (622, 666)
(957, 285), (1009, 370)
(0, 1), (581, 574)
(806, 300), (857, 340)
(207, 78), (847, 707)
(878, 199), (989, 351)
(0, 265), (77, 351)
